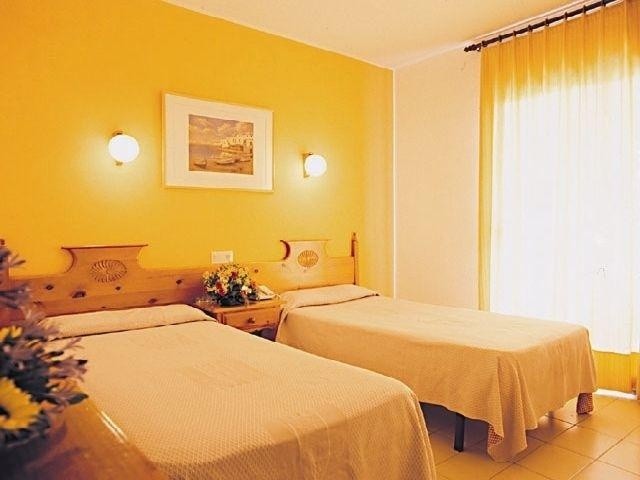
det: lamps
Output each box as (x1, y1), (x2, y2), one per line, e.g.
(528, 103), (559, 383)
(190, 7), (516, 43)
(302, 150), (330, 181)
(108, 129), (140, 168)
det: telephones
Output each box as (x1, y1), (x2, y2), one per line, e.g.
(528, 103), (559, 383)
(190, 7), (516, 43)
(258, 286), (275, 300)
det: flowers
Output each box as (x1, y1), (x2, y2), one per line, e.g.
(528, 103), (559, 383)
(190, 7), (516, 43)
(202, 262), (262, 302)
(0, 237), (88, 439)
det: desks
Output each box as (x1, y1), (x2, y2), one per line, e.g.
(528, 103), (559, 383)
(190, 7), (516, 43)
(0, 399), (178, 480)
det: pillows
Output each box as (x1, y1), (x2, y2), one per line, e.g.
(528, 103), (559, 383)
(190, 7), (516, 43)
(42, 304), (211, 342)
(278, 282), (379, 310)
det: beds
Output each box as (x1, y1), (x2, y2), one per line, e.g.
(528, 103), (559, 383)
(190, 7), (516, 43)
(278, 233), (599, 453)
(41, 243), (438, 480)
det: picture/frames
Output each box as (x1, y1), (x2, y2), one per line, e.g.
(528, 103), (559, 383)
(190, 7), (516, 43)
(162, 89), (276, 195)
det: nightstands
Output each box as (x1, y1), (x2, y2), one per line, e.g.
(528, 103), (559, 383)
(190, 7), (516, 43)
(195, 295), (287, 331)
